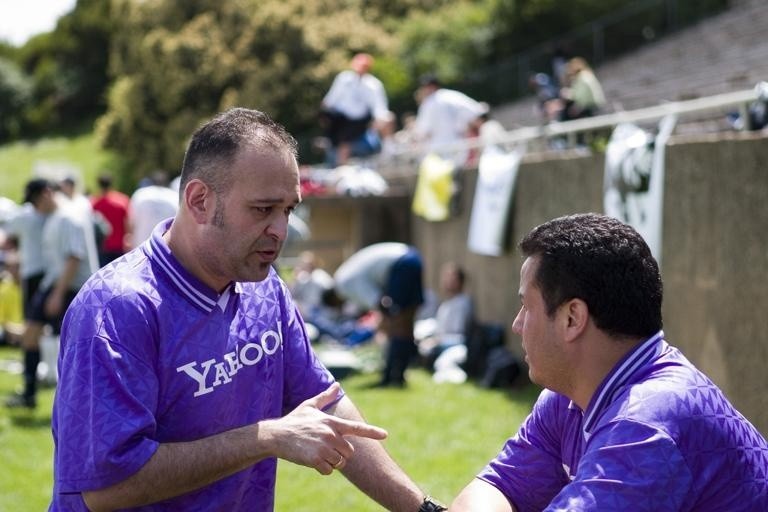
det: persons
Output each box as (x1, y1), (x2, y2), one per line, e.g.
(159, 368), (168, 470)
(2, 170), (182, 407)
(532, 55), (609, 153)
(444, 210), (767, 511)
(44, 105), (444, 512)
(311, 46), (511, 168)
(277, 216), (471, 389)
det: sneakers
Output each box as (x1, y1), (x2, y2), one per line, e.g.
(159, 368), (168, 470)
(4, 396), (37, 409)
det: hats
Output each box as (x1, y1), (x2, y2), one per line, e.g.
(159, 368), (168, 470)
(19, 178), (49, 206)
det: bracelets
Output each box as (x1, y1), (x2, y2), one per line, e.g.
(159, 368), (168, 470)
(419, 494), (449, 511)
